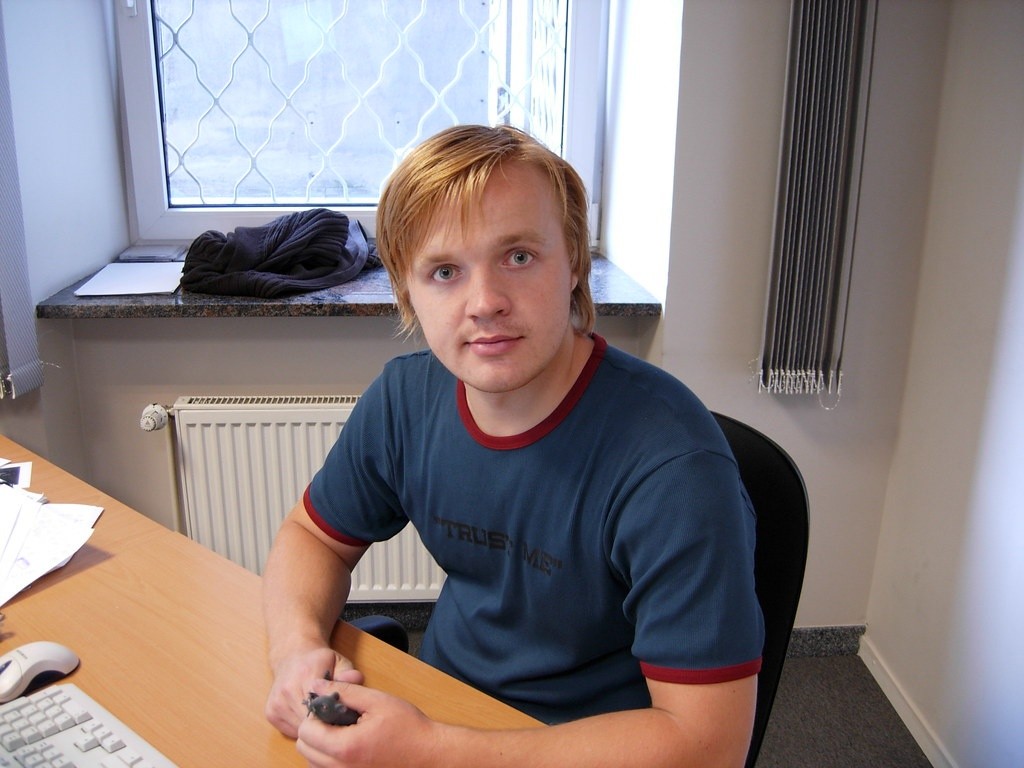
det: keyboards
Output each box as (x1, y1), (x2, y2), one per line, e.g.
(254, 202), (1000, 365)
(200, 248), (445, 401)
(0, 684), (179, 768)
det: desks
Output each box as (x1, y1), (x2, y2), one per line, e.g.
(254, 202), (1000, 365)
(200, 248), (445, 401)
(0, 429), (549, 768)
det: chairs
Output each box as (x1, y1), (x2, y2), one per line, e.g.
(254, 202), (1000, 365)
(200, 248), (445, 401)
(712, 410), (811, 768)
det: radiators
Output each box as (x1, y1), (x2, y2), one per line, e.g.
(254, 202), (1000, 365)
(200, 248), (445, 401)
(143, 394), (447, 603)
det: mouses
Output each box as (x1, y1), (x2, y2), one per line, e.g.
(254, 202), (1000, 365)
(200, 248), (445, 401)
(0, 642), (80, 704)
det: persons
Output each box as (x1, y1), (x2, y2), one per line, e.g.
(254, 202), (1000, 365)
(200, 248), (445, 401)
(263, 124), (765, 768)
